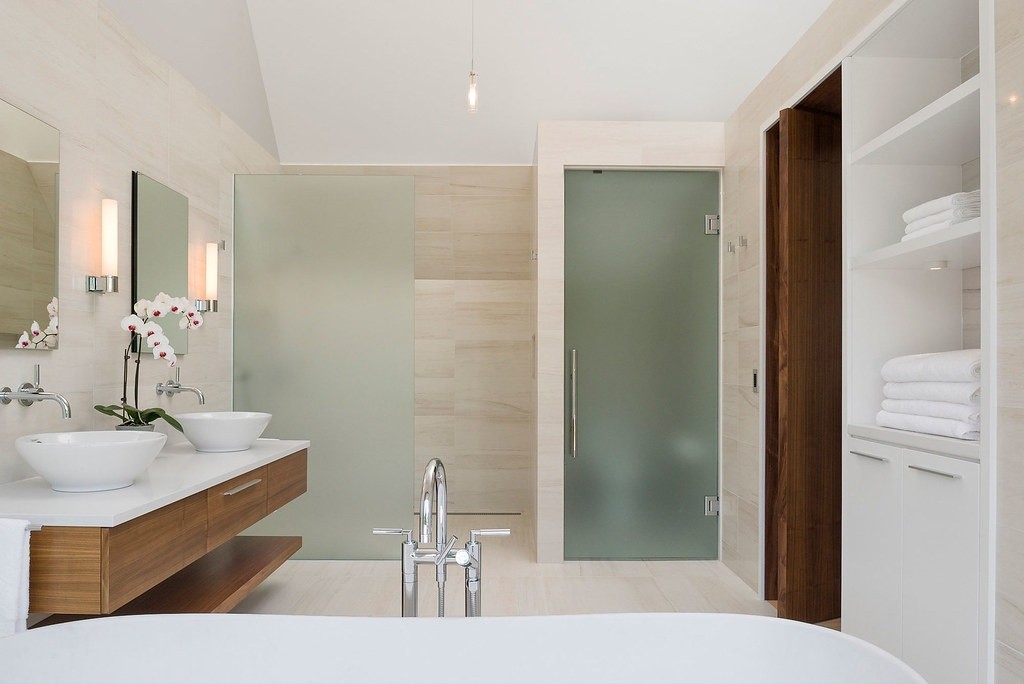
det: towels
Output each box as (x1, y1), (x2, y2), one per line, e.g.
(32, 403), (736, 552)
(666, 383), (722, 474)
(0, 518), (30, 635)
(900, 189), (981, 242)
(875, 349), (981, 441)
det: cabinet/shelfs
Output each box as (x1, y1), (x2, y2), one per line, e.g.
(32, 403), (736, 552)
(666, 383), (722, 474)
(0, 450), (309, 642)
(841, 1), (1024, 684)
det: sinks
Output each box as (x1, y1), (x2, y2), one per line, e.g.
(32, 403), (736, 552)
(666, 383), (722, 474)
(14, 430), (168, 493)
(170, 411), (273, 452)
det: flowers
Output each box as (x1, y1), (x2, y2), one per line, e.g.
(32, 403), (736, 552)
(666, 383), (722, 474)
(15, 296), (58, 350)
(94, 292), (205, 433)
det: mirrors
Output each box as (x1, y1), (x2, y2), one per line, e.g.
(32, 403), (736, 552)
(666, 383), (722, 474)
(135, 170), (190, 356)
(0, 100), (59, 350)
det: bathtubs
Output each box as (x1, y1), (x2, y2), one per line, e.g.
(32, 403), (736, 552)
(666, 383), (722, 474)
(0, 612), (926, 684)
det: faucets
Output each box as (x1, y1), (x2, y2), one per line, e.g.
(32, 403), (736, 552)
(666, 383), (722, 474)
(418, 457), (448, 544)
(18, 382), (71, 420)
(164, 380), (205, 405)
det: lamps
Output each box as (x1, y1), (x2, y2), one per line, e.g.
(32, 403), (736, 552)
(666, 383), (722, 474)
(87, 198), (119, 295)
(195, 242), (219, 312)
(468, 0), (477, 113)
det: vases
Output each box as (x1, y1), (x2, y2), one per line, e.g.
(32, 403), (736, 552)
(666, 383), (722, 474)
(115, 423), (155, 432)
